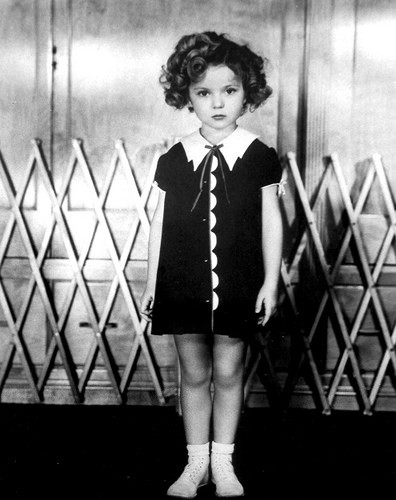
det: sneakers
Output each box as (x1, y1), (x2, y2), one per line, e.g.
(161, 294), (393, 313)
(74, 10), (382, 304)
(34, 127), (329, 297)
(210, 451), (244, 496)
(166, 455), (209, 498)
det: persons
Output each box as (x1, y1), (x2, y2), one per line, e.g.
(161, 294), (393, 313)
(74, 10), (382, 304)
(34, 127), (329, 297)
(139, 29), (288, 498)
